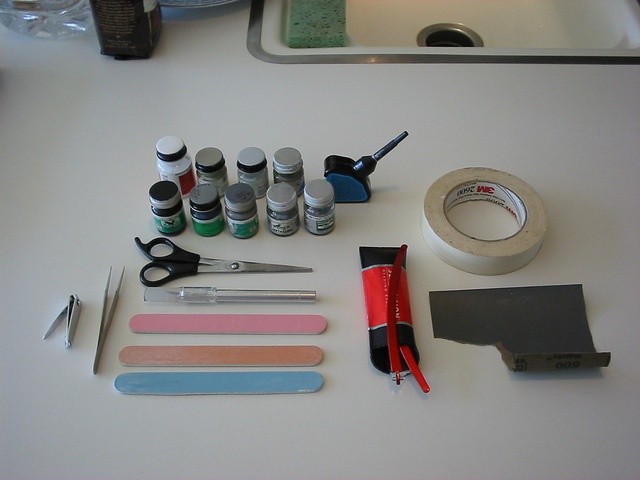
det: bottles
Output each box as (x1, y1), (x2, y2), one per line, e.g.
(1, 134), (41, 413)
(189, 184), (226, 237)
(194, 148), (229, 197)
(224, 183), (258, 239)
(304, 179), (335, 236)
(266, 182), (299, 236)
(155, 136), (196, 198)
(237, 147), (269, 198)
(272, 147), (305, 197)
(148, 181), (186, 237)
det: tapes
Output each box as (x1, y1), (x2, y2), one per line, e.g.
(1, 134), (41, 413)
(419, 167), (548, 276)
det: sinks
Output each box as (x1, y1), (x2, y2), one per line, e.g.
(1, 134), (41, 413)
(261, 0), (640, 55)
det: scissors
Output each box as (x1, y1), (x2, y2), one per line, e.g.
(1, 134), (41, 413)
(135, 237), (313, 286)
